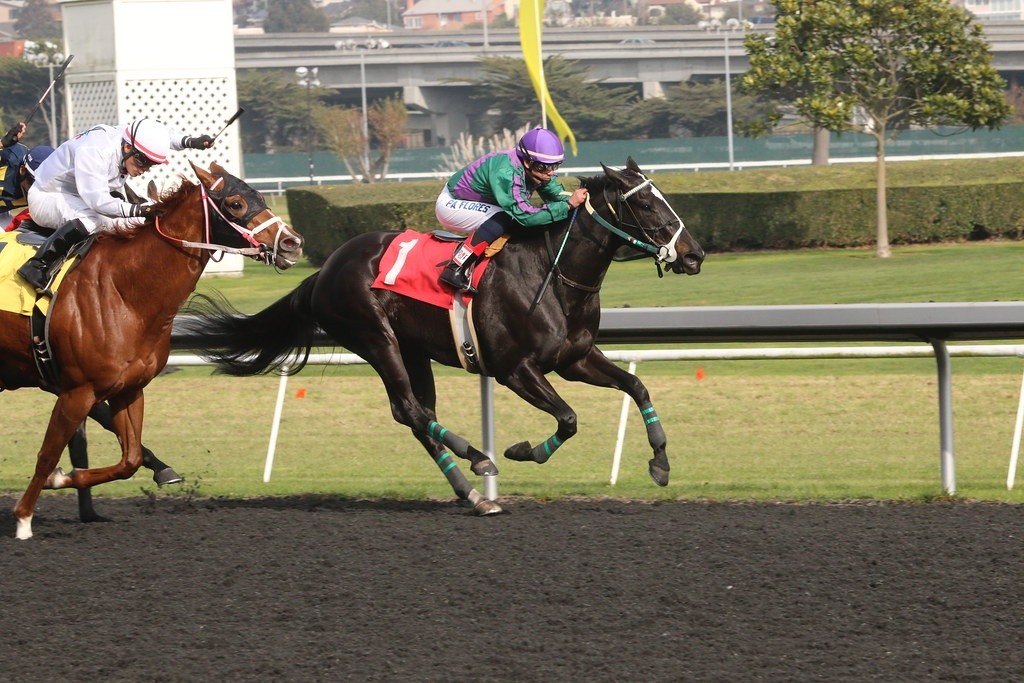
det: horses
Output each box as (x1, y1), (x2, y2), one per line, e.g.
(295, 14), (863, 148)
(177, 155), (706, 515)
(0, 160), (305, 542)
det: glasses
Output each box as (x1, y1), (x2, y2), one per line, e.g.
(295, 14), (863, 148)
(519, 140), (563, 174)
(130, 146), (157, 167)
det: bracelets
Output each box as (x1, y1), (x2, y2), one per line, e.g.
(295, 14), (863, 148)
(566, 201), (575, 212)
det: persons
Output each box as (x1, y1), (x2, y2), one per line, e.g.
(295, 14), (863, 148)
(0, 121), (55, 213)
(436, 129), (588, 293)
(17, 119), (215, 290)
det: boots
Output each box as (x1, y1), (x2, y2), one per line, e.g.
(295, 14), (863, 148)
(440, 240), (480, 295)
(17, 220), (88, 299)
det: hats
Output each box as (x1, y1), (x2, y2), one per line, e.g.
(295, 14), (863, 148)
(122, 119), (170, 164)
(516, 128), (565, 164)
(22, 146), (55, 183)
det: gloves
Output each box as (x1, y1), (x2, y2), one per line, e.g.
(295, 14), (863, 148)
(140, 205), (164, 223)
